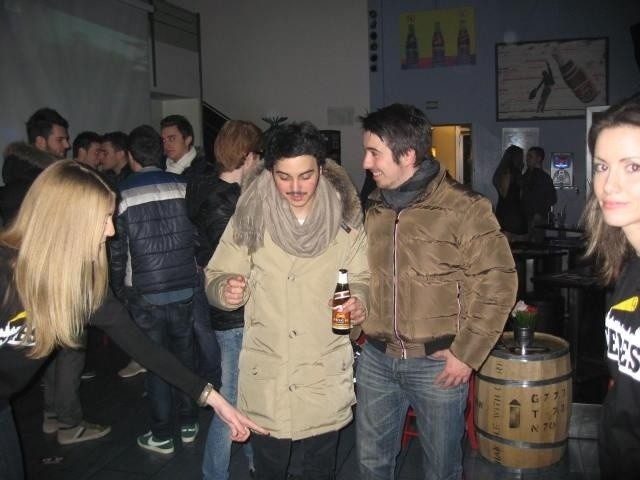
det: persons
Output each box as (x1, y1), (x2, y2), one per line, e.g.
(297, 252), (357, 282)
(337, 102), (517, 480)
(200, 121), (372, 480)
(493, 145), (526, 246)
(526, 60), (559, 116)
(0, 105), (274, 479)
(518, 147), (558, 245)
(570, 94), (640, 478)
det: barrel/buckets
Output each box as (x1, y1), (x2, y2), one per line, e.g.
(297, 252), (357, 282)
(472, 330), (571, 471)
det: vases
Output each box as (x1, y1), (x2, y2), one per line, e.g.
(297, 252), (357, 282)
(515, 326), (534, 347)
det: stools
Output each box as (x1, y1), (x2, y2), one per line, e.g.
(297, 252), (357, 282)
(530, 268), (606, 396)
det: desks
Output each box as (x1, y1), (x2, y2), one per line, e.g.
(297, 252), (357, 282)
(505, 220), (598, 294)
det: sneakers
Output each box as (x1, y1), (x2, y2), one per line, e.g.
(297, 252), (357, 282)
(118, 362), (146, 377)
(58, 418), (111, 445)
(180, 423), (199, 443)
(137, 430), (174, 454)
(43, 412), (59, 434)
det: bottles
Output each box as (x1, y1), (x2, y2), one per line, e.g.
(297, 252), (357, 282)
(333, 270), (351, 333)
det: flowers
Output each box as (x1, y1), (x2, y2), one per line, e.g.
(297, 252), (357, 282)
(511, 300), (536, 329)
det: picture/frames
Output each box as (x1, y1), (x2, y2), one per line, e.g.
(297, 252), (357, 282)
(495, 36), (612, 202)
